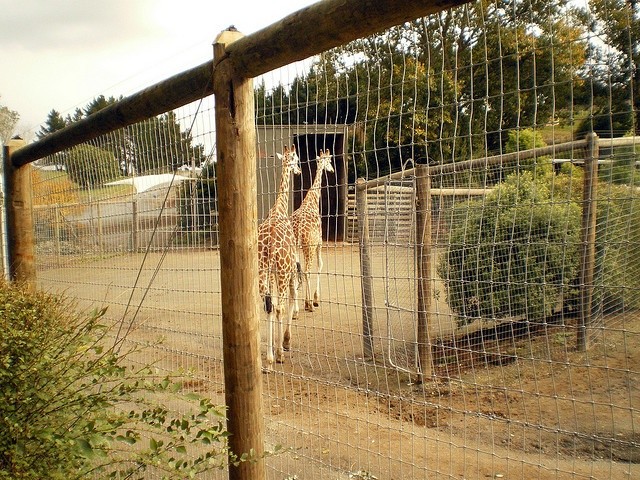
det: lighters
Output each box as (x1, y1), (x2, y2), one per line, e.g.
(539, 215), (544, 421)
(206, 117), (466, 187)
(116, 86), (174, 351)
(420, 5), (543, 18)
(257, 144), (301, 373)
(289, 148), (334, 320)
